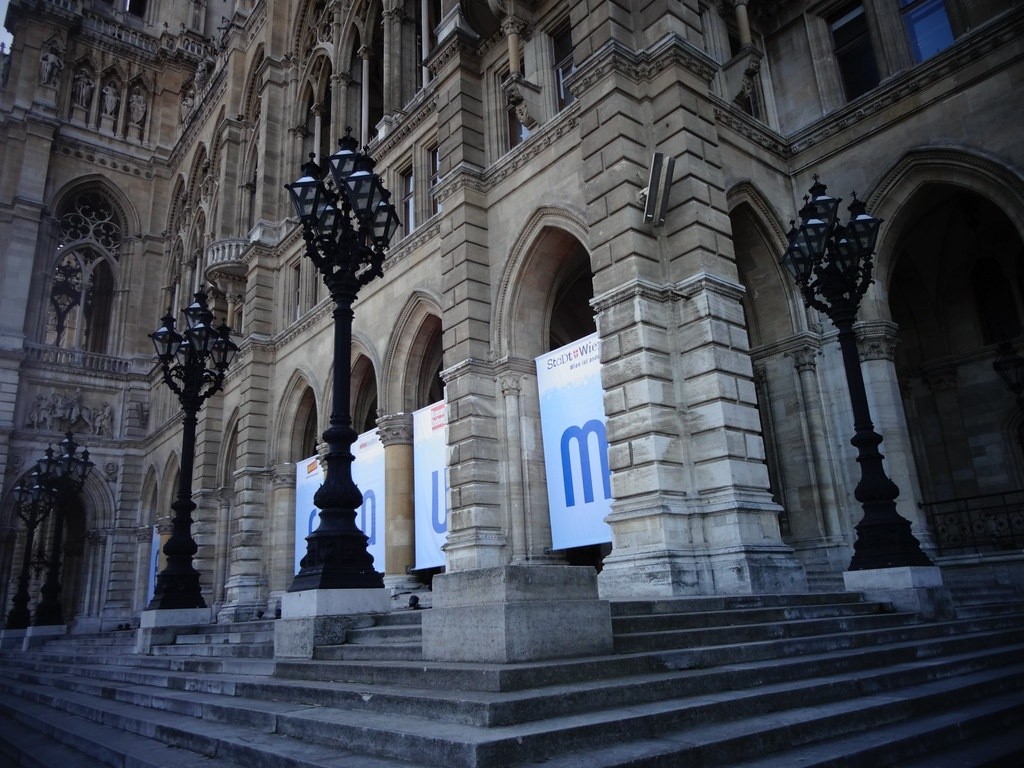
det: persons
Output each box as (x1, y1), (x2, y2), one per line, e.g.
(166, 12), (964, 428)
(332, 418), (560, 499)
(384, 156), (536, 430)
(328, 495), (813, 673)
(24, 387), (114, 436)
(102, 79), (120, 117)
(129, 86), (147, 125)
(181, 95), (194, 122)
(39, 47), (65, 88)
(0, 54), (10, 92)
(72, 67), (94, 109)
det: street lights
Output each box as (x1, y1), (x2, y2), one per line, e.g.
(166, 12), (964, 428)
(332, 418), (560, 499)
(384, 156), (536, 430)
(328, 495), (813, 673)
(289, 120), (403, 590)
(34, 423), (99, 627)
(2, 477), (50, 628)
(143, 281), (244, 610)
(783, 170), (938, 573)
